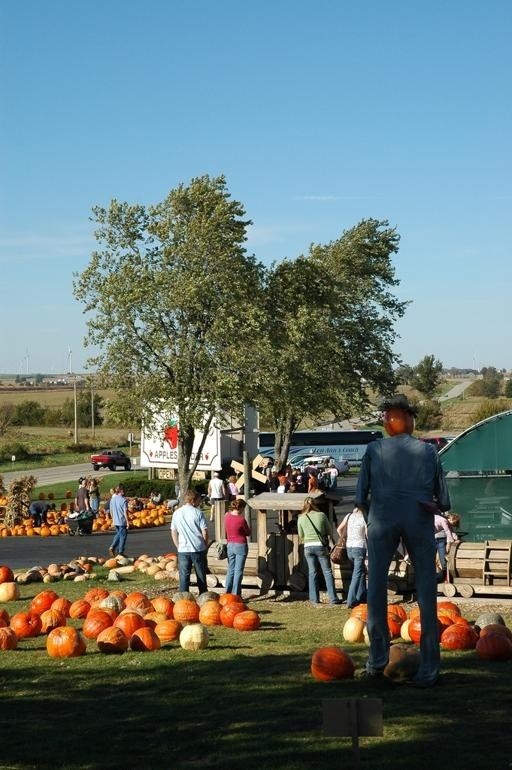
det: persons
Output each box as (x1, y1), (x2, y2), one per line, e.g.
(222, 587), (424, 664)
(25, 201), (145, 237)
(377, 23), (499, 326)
(296, 497), (460, 609)
(109, 483), (130, 557)
(170, 490), (210, 595)
(216, 499), (252, 596)
(28, 473), (179, 527)
(206, 459), (339, 522)
(355, 395), (451, 687)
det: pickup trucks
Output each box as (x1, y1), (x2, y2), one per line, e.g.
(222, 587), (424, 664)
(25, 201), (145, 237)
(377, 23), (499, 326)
(89, 449), (131, 472)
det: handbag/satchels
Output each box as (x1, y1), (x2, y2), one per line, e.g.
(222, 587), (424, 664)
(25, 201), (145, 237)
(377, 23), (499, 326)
(330, 545), (349, 564)
(216, 544), (228, 560)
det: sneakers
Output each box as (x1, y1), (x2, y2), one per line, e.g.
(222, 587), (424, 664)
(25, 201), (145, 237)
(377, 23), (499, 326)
(109, 546), (114, 557)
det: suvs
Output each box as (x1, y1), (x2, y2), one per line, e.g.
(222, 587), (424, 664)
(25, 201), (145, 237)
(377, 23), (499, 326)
(417, 437), (449, 452)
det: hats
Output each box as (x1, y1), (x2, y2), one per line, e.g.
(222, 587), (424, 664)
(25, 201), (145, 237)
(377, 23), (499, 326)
(378, 394), (418, 417)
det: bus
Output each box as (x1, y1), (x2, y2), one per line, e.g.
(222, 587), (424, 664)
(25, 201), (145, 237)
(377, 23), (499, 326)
(256, 429), (384, 469)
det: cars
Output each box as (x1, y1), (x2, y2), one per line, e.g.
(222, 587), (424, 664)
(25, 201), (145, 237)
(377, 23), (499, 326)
(289, 454), (350, 488)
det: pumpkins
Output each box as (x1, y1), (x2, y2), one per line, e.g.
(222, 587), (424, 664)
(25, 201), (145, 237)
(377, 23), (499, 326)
(0, 495), (169, 537)
(0, 588), (262, 658)
(312, 603), (512, 681)
(0, 552), (181, 604)
